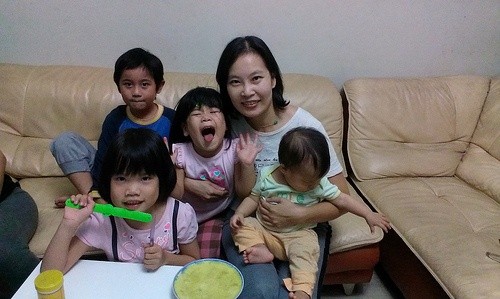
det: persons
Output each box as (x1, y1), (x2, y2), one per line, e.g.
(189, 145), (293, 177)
(0, 153), (39, 299)
(39, 35), (392, 299)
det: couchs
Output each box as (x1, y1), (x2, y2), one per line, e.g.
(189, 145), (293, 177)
(343, 76), (500, 299)
(0, 64), (384, 296)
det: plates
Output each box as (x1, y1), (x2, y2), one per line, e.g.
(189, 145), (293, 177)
(173, 259), (245, 299)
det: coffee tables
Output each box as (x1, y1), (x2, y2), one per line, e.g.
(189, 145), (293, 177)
(11, 259), (184, 299)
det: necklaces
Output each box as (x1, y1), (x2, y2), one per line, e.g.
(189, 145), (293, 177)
(256, 121), (277, 128)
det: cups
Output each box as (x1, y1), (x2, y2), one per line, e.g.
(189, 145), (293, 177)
(34, 270), (64, 299)
